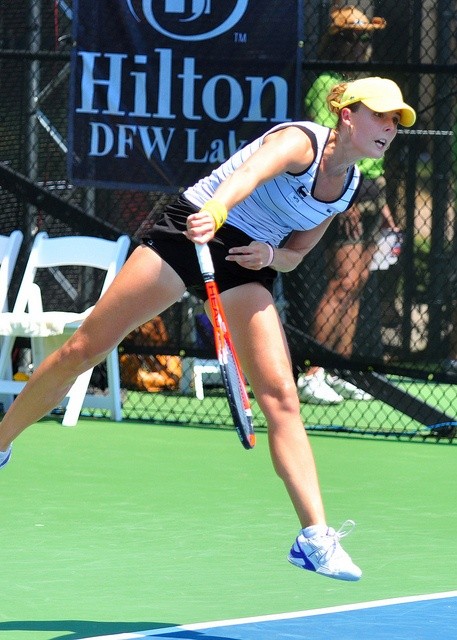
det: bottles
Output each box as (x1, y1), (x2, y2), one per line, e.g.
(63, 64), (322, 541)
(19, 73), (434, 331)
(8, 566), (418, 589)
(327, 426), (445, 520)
(13, 365), (31, 381)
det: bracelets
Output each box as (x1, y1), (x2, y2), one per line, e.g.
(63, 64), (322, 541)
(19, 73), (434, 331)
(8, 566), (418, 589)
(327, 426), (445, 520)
(263, 240), (277, 272)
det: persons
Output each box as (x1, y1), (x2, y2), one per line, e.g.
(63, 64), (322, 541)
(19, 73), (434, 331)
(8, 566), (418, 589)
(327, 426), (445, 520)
(1, 75), (417, 582)
(296, 8), (401, 407)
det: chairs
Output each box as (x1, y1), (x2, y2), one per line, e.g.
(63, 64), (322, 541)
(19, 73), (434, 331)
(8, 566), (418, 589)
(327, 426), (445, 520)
(186, 270), (288, 400)
(1, 230), (24, 311)
(0, 233), (131, 422)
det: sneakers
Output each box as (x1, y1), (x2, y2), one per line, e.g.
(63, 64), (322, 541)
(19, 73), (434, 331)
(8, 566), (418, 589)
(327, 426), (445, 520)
(297, 367), (344, 405)
(326, 372), (375, 400)
(0, 441), (13, 468)
(288, 520), (362, 581)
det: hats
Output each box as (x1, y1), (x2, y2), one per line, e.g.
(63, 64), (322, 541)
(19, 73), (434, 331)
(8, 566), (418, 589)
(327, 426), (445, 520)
(328, 5), (387, 34)
(330, 76), (416, 127)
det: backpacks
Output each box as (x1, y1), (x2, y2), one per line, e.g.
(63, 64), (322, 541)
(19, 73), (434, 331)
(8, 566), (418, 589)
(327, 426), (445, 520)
(119, 316), (181, 393)
